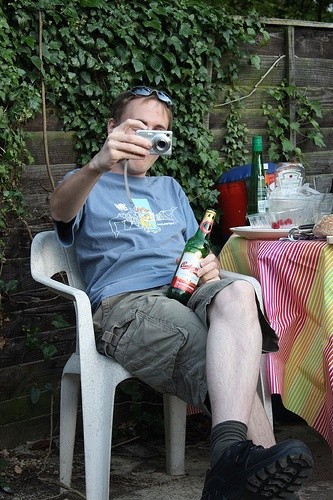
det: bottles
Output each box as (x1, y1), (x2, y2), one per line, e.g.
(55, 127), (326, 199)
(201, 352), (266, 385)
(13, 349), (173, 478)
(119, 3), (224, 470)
(166, 207), (217, 306)
(245, 134), (268, 225)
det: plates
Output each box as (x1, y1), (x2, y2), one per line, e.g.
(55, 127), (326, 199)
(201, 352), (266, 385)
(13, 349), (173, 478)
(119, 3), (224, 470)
(228, 226), (312, 240)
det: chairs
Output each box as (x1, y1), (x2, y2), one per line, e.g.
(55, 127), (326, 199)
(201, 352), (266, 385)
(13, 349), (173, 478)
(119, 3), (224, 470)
(30, 232), (275, 500)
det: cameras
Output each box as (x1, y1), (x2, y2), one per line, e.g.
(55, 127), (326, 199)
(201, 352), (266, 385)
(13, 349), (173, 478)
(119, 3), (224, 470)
(135, 130), (173, 155)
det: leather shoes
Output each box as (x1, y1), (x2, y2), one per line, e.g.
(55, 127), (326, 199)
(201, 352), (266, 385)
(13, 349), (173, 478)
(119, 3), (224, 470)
(200, 439), (313, 500)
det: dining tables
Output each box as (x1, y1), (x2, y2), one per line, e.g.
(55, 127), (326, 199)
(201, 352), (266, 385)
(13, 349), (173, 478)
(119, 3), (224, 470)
(186, 233), (333, 451)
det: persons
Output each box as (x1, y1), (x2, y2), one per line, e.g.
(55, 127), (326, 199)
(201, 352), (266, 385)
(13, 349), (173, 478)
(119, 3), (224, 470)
(49, 86), (313, 500)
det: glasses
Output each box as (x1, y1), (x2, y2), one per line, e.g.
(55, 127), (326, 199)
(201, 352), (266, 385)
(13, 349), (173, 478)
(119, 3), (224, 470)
(124, 86), (174, 109)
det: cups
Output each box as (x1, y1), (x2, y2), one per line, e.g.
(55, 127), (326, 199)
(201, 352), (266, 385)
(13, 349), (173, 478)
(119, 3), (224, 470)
(246, 191), (333, 228)
